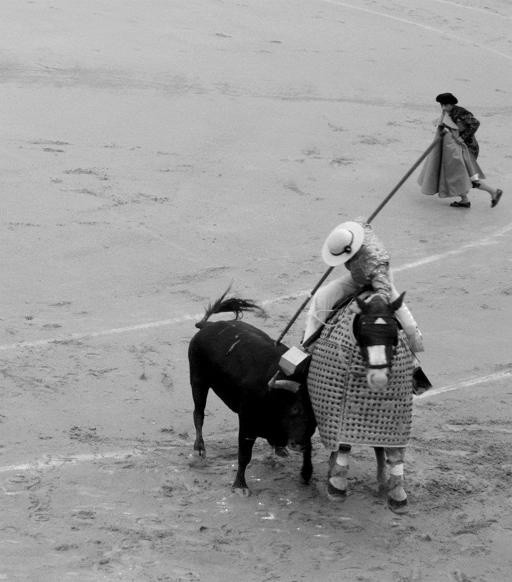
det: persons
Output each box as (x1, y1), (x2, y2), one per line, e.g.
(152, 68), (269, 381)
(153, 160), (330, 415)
(299, 216), (427, 367)
(434, 93), (503, 211)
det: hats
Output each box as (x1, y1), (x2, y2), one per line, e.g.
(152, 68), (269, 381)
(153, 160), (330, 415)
(322, 221), (364, 266)
(436, 93), (457, 104)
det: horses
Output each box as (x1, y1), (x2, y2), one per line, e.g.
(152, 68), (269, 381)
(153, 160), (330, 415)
(324, 291), (410, 514)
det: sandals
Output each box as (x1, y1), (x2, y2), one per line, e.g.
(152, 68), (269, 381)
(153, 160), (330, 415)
(491, 189), (502, 208)
(450, 201), (470, 208)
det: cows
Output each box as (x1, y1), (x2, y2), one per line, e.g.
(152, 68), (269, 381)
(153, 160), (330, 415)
(186, 287), (318, 499)
